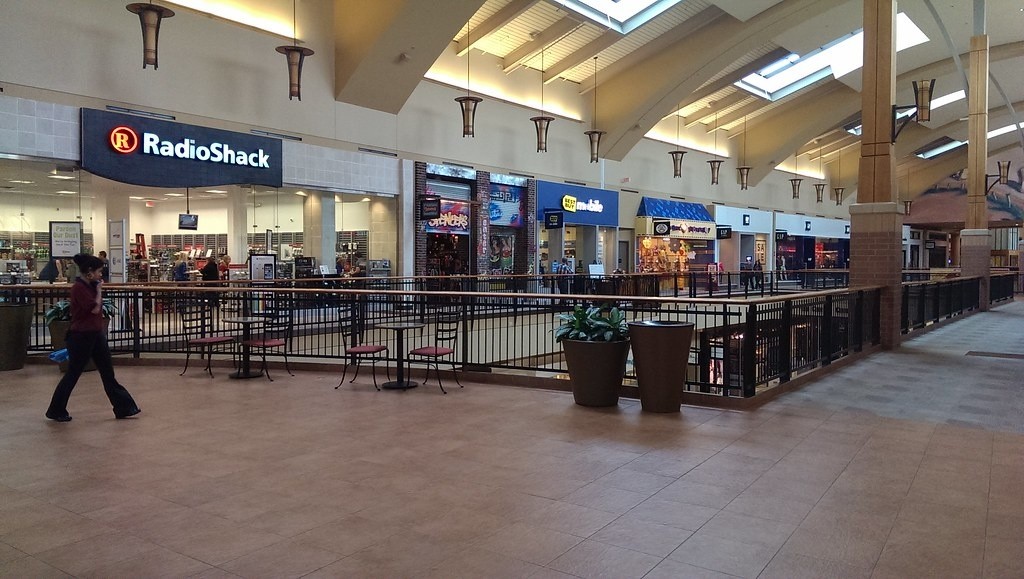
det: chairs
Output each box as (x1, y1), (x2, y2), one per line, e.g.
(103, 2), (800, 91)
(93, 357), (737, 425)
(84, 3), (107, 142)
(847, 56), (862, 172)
(404, 308), (464, 394)
(334, 305), (391, 391)
(234, 302), (297, 382)
(178, 300), (236, 378)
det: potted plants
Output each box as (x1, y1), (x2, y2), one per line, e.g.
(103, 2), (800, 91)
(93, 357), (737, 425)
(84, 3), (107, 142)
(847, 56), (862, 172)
(549, 302), (631, 408)
(44, 297), (117, 373)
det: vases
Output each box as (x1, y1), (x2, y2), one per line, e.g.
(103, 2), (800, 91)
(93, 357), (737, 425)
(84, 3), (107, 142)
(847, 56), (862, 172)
(627, 319), (694, 414)
(0, 302), (34, 370)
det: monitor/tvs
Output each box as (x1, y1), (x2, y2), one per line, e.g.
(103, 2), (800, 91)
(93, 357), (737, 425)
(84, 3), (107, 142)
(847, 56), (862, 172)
(179, 214), (198, 230)
(7, 263), (19, 272)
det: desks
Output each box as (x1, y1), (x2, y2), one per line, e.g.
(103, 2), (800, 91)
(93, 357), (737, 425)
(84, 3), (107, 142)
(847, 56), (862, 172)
(222, 317), (272, 379)
(375, 323), (426, 389)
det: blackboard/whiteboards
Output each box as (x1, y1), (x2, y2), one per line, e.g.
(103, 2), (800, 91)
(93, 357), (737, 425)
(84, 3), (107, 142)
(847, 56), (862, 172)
(49, 220), (82, 258)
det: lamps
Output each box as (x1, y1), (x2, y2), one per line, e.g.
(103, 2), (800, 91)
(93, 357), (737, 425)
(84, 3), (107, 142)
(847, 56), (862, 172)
(454, 20), (483, 139)
(891, 79), (936, 145)
(707, 113), (725, 185)
(276, 1), (313, 101)
(583, 56), (606, 162)
(530, 48), (554, 152)
(901, 167), (914, 216)
(735, 114), (752, 189)
(789, 144), (846, 205)
(667, 102), (687, 177)
(985, 160), (1012, 196)
(126, 0), (177, 71)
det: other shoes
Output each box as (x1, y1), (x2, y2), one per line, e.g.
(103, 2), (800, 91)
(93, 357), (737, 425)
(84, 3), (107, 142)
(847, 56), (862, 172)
(116, 408), (141, 418)
(46, 409), (72, 421)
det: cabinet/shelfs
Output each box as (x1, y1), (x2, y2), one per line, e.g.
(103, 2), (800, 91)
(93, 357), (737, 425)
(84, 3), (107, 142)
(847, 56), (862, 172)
(0, 246), (394, 316)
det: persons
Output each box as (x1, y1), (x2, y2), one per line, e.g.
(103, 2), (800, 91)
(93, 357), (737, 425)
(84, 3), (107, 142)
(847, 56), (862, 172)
(39, 259), (64, 282)
(556, 258), (573, 306)
(198, 255), (231, 306)
(336, 252), (366, 277)
(45, 252), (142, 422)
(98, 251), (109, 283)
(779, 255), (788, 281)
(744, 261), (763, 291)
(173, 252), (190, 287)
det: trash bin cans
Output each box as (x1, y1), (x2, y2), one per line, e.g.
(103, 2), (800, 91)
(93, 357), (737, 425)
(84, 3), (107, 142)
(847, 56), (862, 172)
(626, 320), (697, 413)
(0, 298), (35, 370)
